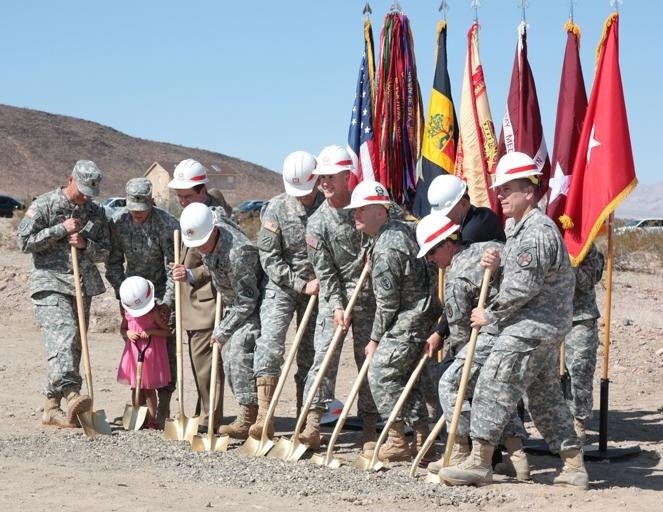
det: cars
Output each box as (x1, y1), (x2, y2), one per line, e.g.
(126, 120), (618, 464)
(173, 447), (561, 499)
(233, 198), (265, 216)
(102, 196), (126, 214)
(0, 193), (25, 219)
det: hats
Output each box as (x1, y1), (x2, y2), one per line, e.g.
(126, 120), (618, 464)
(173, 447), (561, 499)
(124, 178), (153, 212)
(207, 188), (226, 205)
(72, 159), (103, 196)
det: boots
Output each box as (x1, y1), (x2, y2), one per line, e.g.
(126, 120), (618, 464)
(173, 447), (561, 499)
(496, 438), (530, 480)
(249, 376), (279, 440)
(362, 413), (411, 460)
(427, 436), (471, 473)
(42, 385), (94, 428)
(574, 418), (586, 442)
(158, 390), (171, 429)
(441, 439), (494, 485)
(218, 403), (258, 439)
(296, 383), (324, 450)
(411, 424), (436, 457)
(535, 449), (589, 490)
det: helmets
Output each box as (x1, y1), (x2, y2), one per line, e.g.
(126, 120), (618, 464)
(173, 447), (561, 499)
(283, 150), (320, 198)
(342, 180), (390, 210)
(415, 213), (461, 259)
(119, 276), (156, 318)
(426, 175), (467, 215)
(312, 144), (356, 175)
(179, 201), (216, 247)
(488, 152), (543, 189)
(168, 158), (209, 190)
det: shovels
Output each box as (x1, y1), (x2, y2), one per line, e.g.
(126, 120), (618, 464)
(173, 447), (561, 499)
(309, 356), (373, 468)
(163, 229), (200, 447)
(193, 291), (230, 453)
(400, 416), (446, 480)
(65, 220), (113, 442)
(425, 266), (492, 484)
(123, 333), (151, 434)
(353, 354), (430, 472)
(238, 294), (316, 458)
(267, 263), (371, 460)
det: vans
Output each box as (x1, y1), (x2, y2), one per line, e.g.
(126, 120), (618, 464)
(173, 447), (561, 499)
(614, 217), (663, 237)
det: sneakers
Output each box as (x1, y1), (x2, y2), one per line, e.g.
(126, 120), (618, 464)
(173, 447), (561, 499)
(198, 423), (217, 434)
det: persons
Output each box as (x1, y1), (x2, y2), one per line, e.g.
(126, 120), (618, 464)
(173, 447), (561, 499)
(15, 142), (601, 488)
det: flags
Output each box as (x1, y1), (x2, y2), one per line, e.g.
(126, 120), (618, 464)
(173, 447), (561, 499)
(346, 10), (638, 270)
